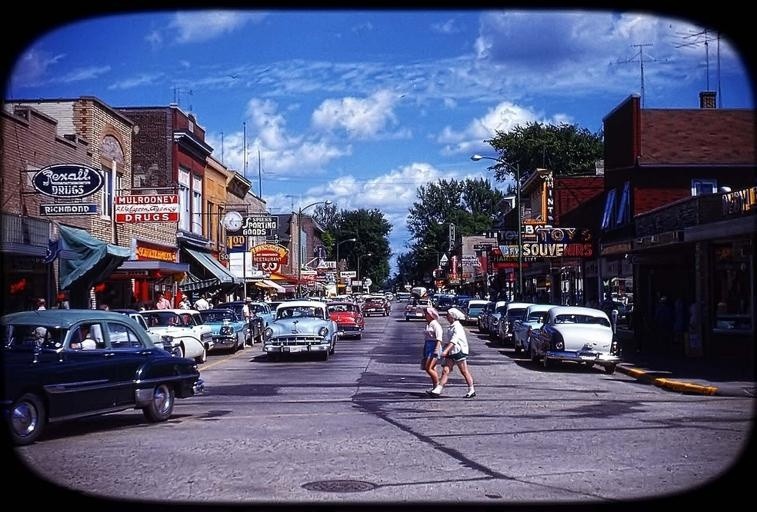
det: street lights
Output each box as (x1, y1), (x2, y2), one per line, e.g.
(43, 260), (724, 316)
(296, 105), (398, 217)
(357, 252), (372, 290)
(437, 220), (454, 228)
(336, 236), (356, 262)
(470, 152), (524, 296)
(296, 199), (332, 292)
(422, 245), (440, 268)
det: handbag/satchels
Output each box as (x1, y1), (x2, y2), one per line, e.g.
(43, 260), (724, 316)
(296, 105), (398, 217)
(421, 358), (427, 370)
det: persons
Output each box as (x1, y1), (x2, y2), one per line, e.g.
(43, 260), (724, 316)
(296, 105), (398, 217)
(183, 314), (191, 326)
(423, 308), (443, 396)
(193, 294), (209, 311)
(70, 325), (96, 349)
(37, 299), (47, 310)
(167, 316), (178, 327)
(155, 291), (171, 309)
(179, 294), (192, 310)
(431, 308), (475, 398)
(243, 296), (254, 349)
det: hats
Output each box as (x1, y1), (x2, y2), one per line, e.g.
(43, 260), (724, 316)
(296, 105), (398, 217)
(427, 308), (439, 320)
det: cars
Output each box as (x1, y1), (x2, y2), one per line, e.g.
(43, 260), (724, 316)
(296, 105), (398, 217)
(510, 303), (576, 356)
(528, 305), (622, 376)
(0, 306), (204, 446)
(397, 288), (530, 344)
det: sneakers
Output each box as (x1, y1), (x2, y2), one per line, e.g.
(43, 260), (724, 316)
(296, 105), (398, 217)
(464, 392), (476, 398)
(426, 385), (442, 396)
